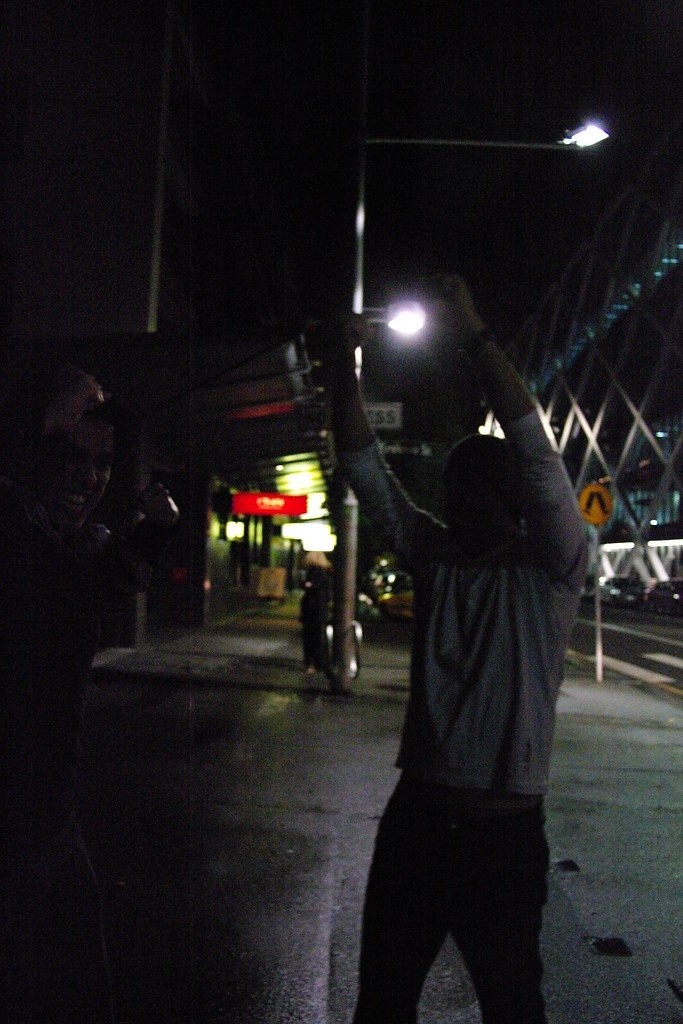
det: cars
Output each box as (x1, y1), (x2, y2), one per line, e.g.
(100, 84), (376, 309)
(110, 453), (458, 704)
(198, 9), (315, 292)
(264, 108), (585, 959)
(361, 559), (416, 620)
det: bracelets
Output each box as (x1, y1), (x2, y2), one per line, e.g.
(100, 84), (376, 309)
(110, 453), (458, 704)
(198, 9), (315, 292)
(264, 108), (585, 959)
(466, 323), (494, 363)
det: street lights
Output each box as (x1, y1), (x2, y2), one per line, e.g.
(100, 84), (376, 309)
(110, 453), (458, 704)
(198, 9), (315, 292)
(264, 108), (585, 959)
(327, 118), (613, 683)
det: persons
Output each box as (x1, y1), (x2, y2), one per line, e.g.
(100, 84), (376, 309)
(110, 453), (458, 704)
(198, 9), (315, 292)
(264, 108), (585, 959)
(0, 361), (183, 1024)
(300, 564), (332, 675)
(313, 265), (593, 1023)
